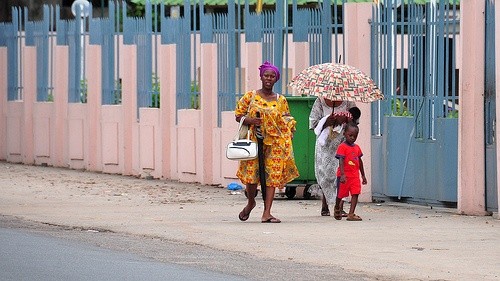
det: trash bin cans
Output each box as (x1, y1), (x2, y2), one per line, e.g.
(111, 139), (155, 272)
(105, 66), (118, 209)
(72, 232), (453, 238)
(284, 94), (319, 200)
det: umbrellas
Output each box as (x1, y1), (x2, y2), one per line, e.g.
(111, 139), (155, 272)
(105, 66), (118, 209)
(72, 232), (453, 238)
(288, 54), (384, 128)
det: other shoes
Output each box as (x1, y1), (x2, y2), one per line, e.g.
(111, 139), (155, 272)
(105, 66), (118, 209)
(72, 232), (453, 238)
(333, 205), (342, 220)
(346, 213), (362, 221)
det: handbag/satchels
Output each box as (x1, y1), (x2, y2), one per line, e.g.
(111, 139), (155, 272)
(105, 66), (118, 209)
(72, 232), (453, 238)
(226, 116), (258, 161)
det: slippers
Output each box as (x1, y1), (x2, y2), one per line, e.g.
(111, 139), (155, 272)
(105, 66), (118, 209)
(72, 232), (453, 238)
(341, 210), (347, 217)
(321, 204), (330, 216)
(261, 217), (281, 223)
(239, 203), (256, 221)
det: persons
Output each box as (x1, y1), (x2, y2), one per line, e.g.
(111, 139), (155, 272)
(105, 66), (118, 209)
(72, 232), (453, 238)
(233, 62), (296, 223)
(309, 95), (368, 220)
